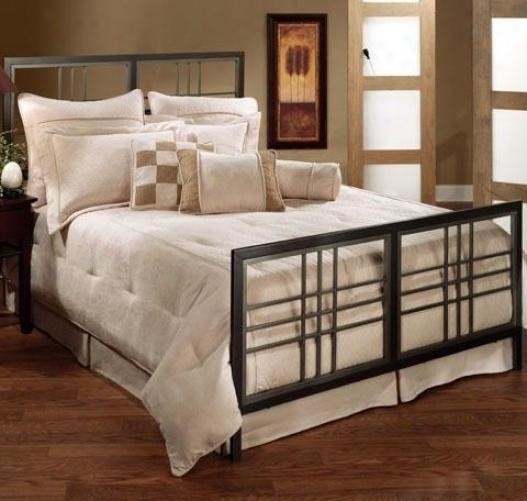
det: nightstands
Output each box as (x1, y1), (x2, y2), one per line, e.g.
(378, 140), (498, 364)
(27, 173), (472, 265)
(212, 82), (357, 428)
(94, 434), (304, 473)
(0, 195), (38, 334)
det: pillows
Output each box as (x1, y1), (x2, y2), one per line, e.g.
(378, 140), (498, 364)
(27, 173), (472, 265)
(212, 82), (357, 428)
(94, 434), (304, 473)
(18, 90), (144, 197)
(144, 113), (260, 151)
(131, 140), (215, 211)
(38, 118), (185, 235)
(148, 92), (258, 116)
(275, 160), (341, 200)
(177, 150), (284, 213)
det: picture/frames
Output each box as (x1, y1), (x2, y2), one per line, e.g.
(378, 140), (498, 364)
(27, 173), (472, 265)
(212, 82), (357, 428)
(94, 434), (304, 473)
(266, 12), (329, 149)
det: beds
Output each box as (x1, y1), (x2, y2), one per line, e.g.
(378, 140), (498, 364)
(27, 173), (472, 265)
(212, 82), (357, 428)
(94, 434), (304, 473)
(4, 52), (522, 455)
